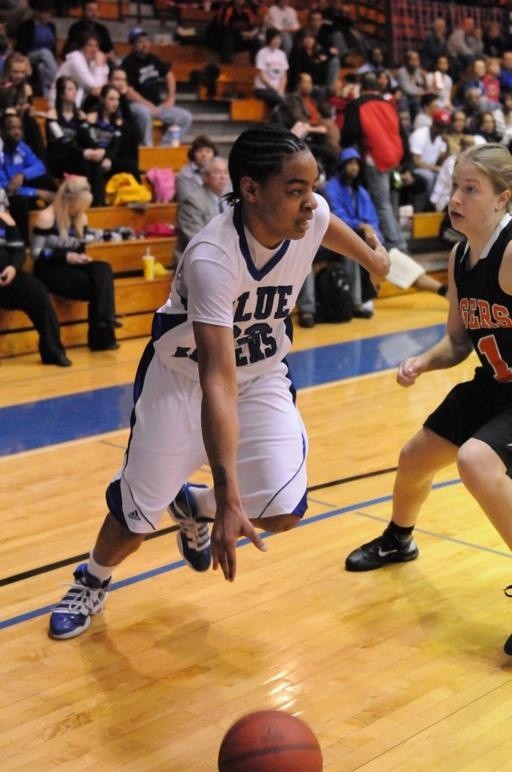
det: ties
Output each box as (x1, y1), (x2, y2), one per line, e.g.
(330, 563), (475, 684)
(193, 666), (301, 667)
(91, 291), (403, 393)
(218, 201), (224, 214)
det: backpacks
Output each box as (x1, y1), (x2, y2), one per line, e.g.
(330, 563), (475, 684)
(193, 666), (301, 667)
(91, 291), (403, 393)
(145, 167), (177, 205)
(102, 171), (153, 208)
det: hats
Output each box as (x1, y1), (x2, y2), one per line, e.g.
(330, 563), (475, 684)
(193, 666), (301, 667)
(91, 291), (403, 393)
(338, 147), (363, 166)
(431, 107), (453, 126)
(127, 27), (148, 44)
(187, 136), (218, 163)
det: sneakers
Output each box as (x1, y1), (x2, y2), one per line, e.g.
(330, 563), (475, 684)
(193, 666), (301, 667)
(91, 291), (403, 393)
(165, 481), (212, 572)
(47, 563), (113, 640)
(344, 528), (419, 573)
(352, 305), (373, 320)
(298, 310), (315, 330)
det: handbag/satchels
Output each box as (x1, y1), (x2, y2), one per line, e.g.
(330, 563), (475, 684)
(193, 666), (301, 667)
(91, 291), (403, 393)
(314, 265), (355, 327)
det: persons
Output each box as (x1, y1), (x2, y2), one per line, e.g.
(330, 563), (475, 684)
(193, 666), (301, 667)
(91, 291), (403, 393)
(346, 143), (512, 657)
(48, 123), (390, 641)
(2, 0), (511, 372)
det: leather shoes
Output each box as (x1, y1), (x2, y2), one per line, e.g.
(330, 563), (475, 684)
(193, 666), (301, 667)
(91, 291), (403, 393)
(41, 352), (71, 367)
(98, 318), (123, 329)
(90, 343), (121, 352)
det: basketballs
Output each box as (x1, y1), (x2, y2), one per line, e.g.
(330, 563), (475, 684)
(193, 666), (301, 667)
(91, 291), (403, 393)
(219, 711), (325, 767)
